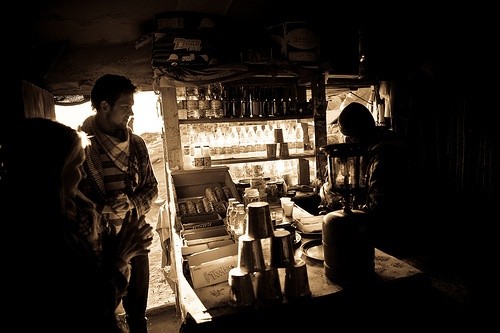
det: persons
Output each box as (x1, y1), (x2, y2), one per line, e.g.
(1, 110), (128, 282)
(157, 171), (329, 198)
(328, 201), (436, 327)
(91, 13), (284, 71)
(0, 82), (153, 333)
(324, 102), (430, 257)
(75, 74), (158, 333)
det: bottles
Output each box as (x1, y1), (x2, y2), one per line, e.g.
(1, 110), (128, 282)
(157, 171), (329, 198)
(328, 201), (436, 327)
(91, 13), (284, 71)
(226, 177), (283, 242)
(177, 82), (314, 120)
(183, 124), (264, 170)
(264, 123), (305, 154)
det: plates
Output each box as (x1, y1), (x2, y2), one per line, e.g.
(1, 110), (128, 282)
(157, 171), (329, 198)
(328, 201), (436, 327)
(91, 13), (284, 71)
(300, 238), (324, 262)
(291, 222), (322, 238)
(293, 232), (302, 245)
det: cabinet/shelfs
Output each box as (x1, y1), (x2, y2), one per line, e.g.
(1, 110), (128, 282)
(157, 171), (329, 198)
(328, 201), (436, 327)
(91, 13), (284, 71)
(178, 113), (317, 166)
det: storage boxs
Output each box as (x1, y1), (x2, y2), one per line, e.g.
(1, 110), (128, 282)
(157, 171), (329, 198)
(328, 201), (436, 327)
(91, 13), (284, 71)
(169, 166), (238, 288)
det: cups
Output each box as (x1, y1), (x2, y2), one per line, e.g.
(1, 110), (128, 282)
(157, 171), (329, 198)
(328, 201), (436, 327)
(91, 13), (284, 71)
(255, 267), (283, 303)
(280, 197), (294, 217)
(283, 260), (312, 300)
(237, 234), (266, 272)
(226, 267), (256, 309)
(267, 144), (277, 158)
(275, 129), (284, 143)
(268, 230), (297, 268)
(280, 143), (289, 157)
(247, 201), (275, 239)
